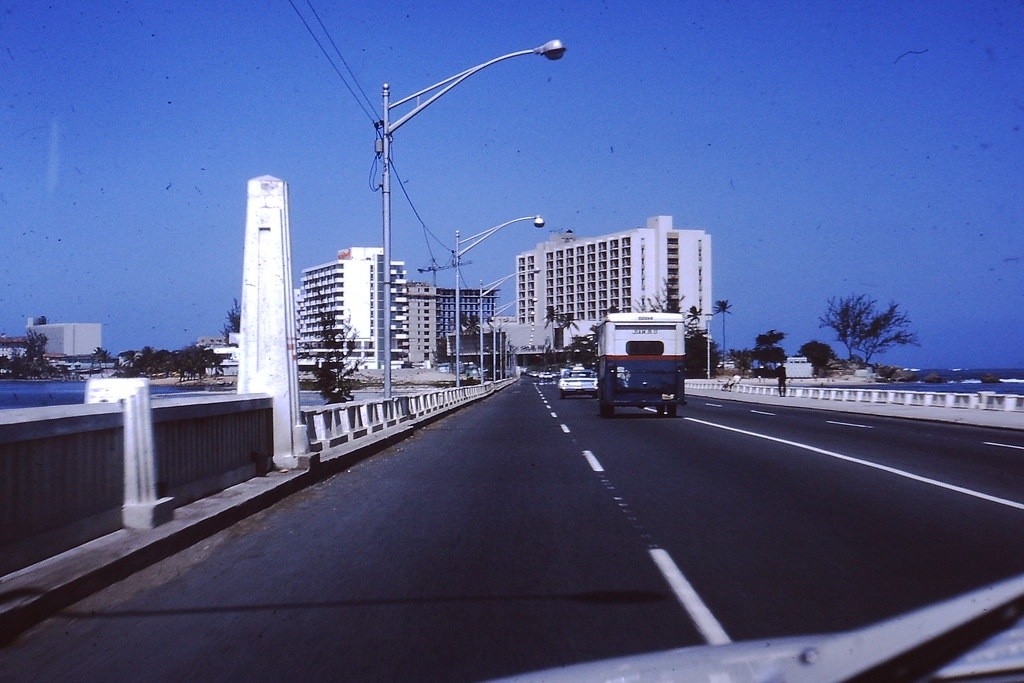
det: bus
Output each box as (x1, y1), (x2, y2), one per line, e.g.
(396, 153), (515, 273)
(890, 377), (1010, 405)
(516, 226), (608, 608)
(590, 312), (687, 419)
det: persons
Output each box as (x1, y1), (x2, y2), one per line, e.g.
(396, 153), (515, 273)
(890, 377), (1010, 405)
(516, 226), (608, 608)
(721, 374), (741, 392)
(775, 361), (787, 397)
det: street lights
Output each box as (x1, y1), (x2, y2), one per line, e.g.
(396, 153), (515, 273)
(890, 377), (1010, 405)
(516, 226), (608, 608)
(456, 215), (544, 388)
(478, 267), (542, 384)
(382, 39), (567, 401)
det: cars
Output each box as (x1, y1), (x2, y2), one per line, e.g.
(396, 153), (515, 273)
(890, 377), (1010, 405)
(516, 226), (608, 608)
(526, 370), (561, 380)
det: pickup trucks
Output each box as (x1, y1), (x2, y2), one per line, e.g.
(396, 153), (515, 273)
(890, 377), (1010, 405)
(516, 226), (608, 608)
(558, 370), (599, 399)
